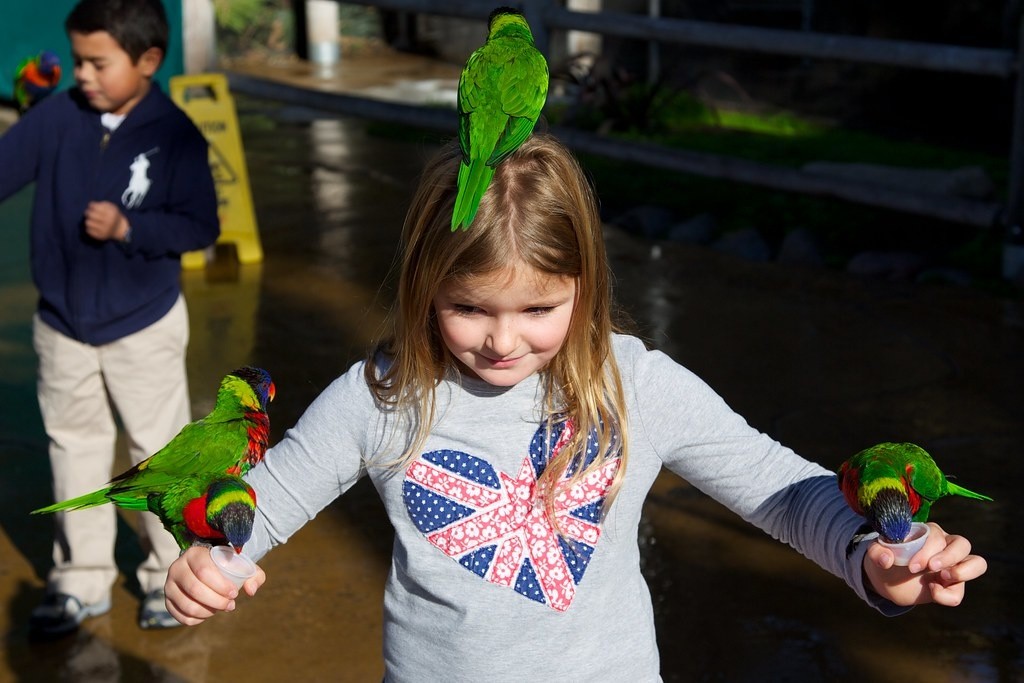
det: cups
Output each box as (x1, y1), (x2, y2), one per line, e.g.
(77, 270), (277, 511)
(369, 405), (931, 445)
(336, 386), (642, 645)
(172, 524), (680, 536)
(210, 546), (256, 589)
(878, 522), (930, 566)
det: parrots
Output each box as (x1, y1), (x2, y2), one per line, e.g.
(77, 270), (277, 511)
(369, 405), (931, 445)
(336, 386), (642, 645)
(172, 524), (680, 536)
(30, 364), (278, 556)
(451, 12), (550, 232)
(838, 443), (994, 546)
(12, 48), (64, 112)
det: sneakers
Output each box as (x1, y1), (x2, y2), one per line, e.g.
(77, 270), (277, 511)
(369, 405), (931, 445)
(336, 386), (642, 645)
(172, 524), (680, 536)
(28, 590), (111, 632)
(140, 589), (186, 628)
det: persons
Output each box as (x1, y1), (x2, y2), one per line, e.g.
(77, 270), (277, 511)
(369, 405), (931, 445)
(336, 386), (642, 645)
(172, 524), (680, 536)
(161, 132), (990, 683)
(0, 1), (223, 641)
